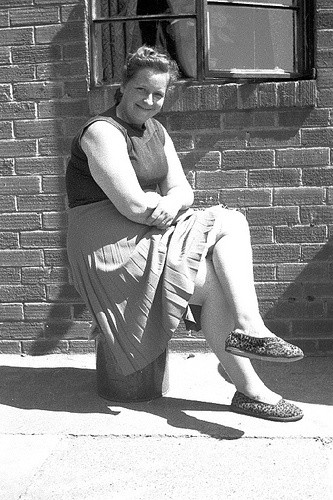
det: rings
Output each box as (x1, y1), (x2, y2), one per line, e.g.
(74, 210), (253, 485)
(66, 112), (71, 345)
(162, 219), (167, 224)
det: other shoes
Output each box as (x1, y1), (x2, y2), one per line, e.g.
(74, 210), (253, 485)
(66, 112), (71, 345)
(223, 332), (304, 362)
(230, 392), (305, 423)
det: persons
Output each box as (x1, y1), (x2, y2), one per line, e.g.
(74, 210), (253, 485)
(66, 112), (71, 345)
(63, 46), (308, 424)
